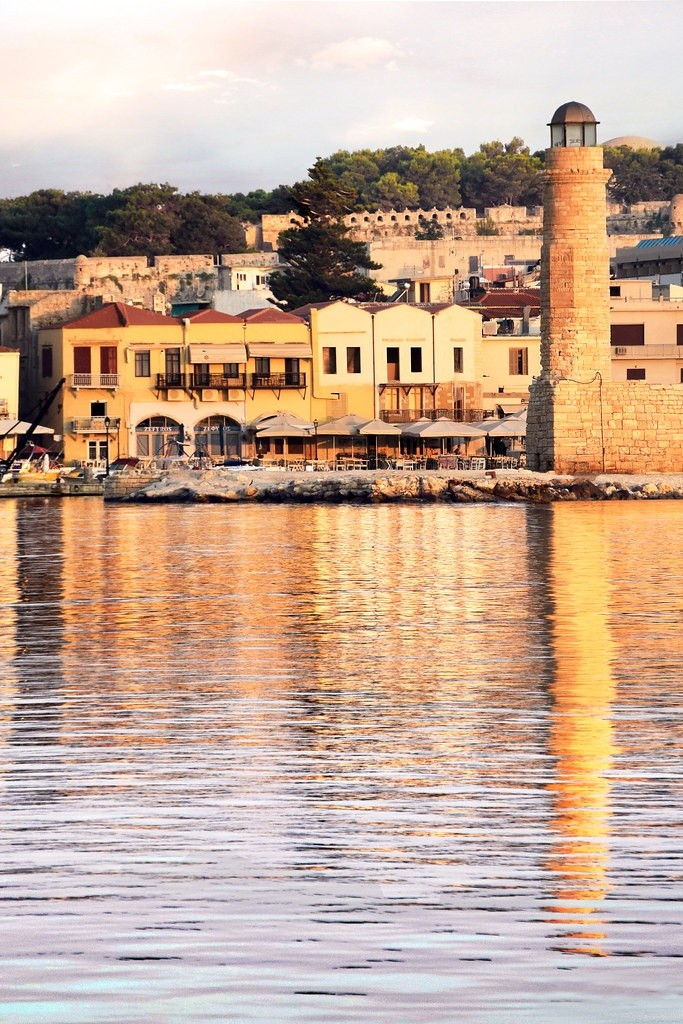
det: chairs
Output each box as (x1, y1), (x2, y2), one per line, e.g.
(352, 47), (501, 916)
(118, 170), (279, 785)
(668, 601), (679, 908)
(258, 457), (518, 472)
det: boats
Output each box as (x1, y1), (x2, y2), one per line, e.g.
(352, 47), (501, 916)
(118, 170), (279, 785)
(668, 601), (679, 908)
(212, 460), (266, 473)
(0, 454), (141, 484)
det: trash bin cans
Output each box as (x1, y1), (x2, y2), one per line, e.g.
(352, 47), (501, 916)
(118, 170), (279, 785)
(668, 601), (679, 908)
(426, 458), (438, 471)
(305, 465), (313, 472)
(253, 459), (260, 466)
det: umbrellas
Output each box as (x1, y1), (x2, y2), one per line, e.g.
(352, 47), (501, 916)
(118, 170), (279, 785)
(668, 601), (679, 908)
(358, 418), (402, 469)
(395, 415), (433, 454)
(410, 415), (487, 455)
(467, 415), (501, 457)
(513, 408), (527, 422)
(316, 412), (372, 469)
(248, 411), (315, 458)
(256, 420), (311, 468)
(19, 446), (59, 459)
(478, 414), (528, 450)
(0, 419), (54, 434)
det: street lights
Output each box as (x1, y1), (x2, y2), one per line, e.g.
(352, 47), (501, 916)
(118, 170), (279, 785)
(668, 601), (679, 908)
(104, 416), (110, 475)
(312, 418), (319, 460)
